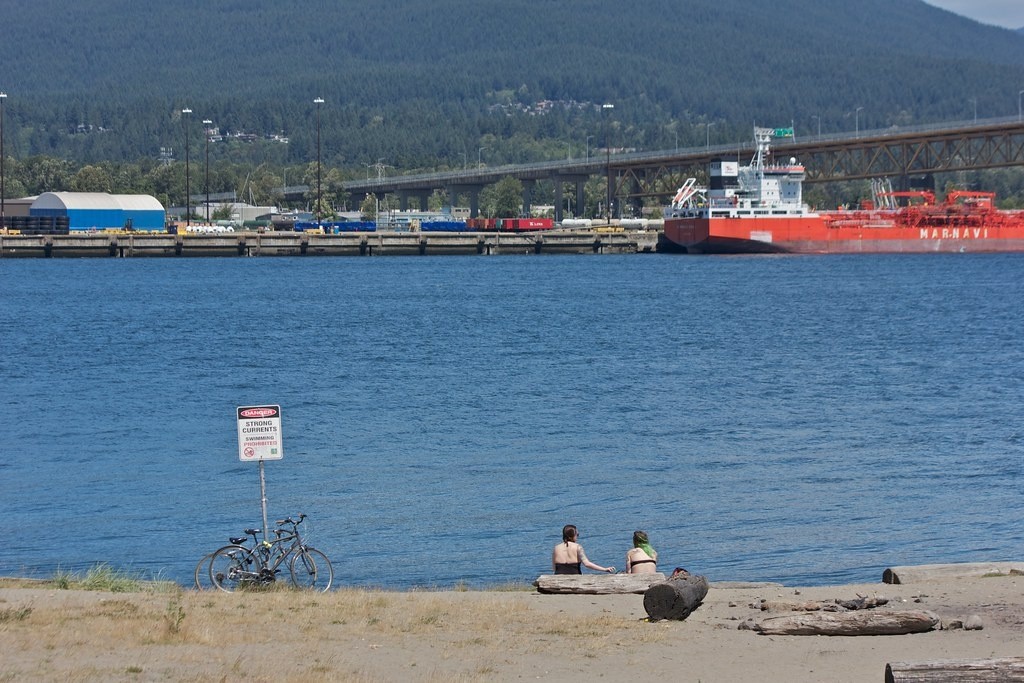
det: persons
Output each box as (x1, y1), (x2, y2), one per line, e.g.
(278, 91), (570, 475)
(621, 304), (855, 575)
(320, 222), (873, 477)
(626, 531), (657, 573)
(552, 525), (615, 573)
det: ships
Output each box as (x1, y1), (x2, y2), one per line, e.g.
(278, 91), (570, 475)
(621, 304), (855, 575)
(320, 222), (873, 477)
(664, 137), (1024, 254)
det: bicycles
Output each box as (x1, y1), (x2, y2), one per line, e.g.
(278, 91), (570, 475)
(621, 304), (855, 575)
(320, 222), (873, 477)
(195, 514), (333, 595)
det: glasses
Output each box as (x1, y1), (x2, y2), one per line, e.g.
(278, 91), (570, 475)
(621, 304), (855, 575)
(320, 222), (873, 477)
(573, 533), (579, 537)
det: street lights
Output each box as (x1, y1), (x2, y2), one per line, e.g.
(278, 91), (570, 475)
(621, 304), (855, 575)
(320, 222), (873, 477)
(203, 119), (213, 219)
(314, 95), (326, 211)
(182, 108), (193, 226)
(0, 91), (7, 218)
